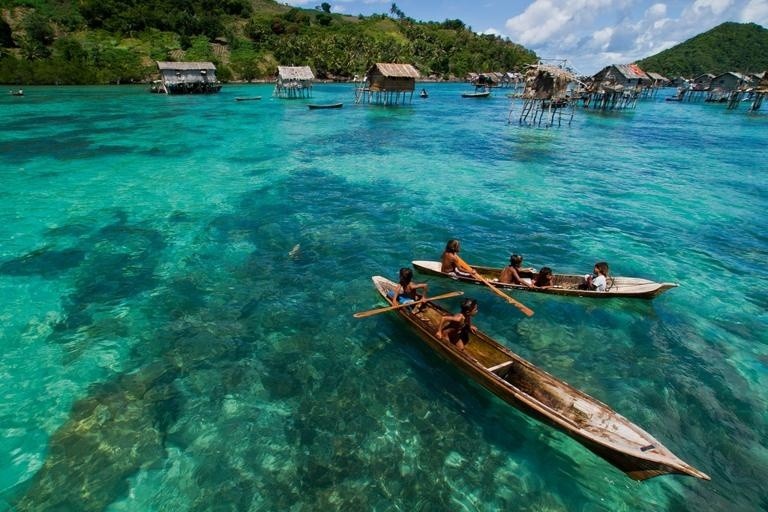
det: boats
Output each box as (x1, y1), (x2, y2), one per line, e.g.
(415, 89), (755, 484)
(9, 89), (24, 96)
(411, 259), (679, 299)
(371, 275), (711, 481)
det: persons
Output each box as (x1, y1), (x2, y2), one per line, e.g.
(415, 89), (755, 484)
(387, 268), (428, 313)
(441, 240), (476, 275)
(532, 267), (554, 290)
(434, 297), (479, 351)
(578, 262), (608, 291)
(499, 255), (534, 289)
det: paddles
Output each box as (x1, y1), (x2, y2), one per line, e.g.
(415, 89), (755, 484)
(456, 257), (535, 317)
(353, 291), (464, 317)
